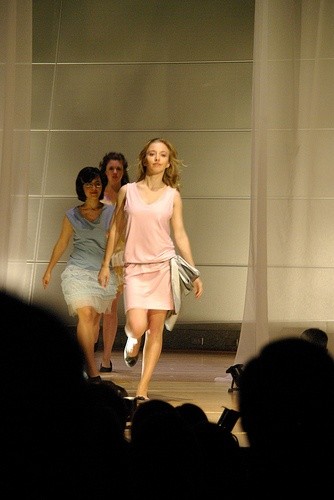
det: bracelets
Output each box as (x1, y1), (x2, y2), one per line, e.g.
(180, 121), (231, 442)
(100, 264), (111, 269)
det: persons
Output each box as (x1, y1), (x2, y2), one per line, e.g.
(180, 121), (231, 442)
(0, 288), (334, 500)
(99, 136), (203, 402)
(93, 152), (131, 373)
(42, 165), (120, 385)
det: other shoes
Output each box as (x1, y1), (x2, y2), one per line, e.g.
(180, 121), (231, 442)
(136, 394), (150, 403)
(90, 376), (101, 384)
(124, 347), (139, 369)
(100, 360), (112, 372)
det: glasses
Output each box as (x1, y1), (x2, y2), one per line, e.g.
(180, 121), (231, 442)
(83, 182), (102, 188)
